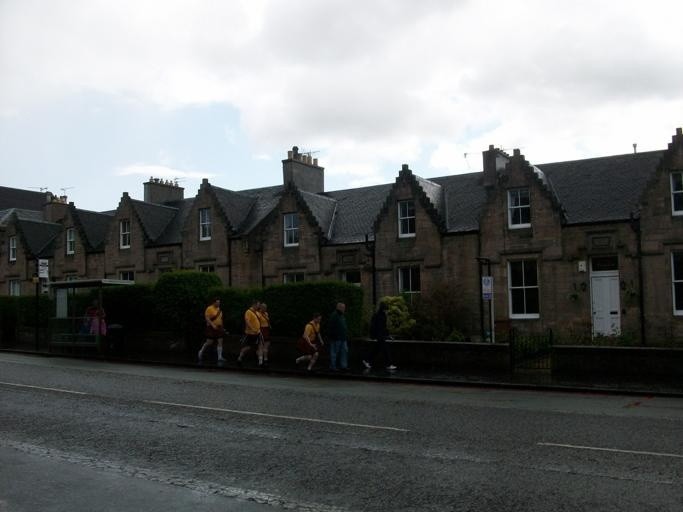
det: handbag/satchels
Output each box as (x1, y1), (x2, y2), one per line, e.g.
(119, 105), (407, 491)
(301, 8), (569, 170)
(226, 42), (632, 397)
(89, 318), (107, 336)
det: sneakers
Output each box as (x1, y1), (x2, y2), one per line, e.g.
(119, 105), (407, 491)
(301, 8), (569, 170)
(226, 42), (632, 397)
(362, 359), (372, 368)
(386, 364), (397, 370)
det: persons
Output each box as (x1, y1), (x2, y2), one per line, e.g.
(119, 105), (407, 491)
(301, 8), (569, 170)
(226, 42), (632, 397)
(294, 314), (326, 370)
(362, 301), (398, 371)
(236, 300), (262, 365)
(198, 298), (230, 361)
(256, 304), (270, 361)
(330, 302), (352, 372)
(84, 298), (106, 336)
